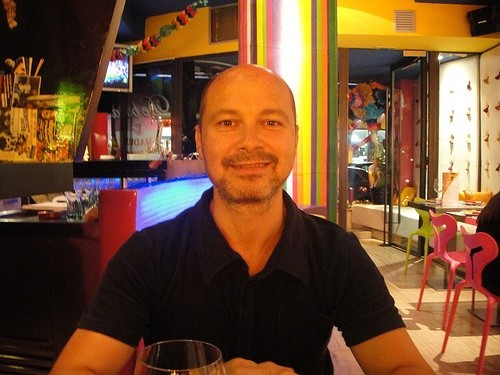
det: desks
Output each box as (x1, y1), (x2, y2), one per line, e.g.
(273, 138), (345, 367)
(446, 211), (500, 327)
(408, 199), (487, 291)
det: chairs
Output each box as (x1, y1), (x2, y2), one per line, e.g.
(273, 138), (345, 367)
(417, 209), (475, 330)
(404, 197), (443, 273)
(441, 225), (500, 375)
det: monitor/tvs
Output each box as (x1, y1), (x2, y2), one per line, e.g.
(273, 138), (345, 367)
(102, 44), (133, 92)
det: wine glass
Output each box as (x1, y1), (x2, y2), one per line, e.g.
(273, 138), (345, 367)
(433, 179), (442, 201)
(73, 177), (158, 212)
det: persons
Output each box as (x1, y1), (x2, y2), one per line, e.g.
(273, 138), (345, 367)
(469, 191), (500, 300)
(50, 64), (434, 375)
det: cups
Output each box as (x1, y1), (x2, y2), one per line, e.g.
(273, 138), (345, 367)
(63, 191), (81, 222)
(138, 340), (226, 375)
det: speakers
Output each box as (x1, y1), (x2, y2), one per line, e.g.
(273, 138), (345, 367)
(467, 5), (500, 36)
